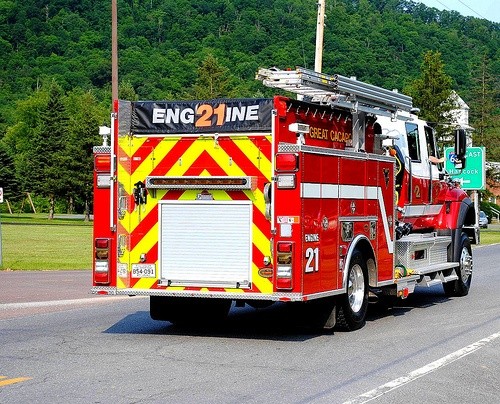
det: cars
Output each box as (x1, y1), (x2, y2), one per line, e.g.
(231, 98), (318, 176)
(479, 211), (489, 229)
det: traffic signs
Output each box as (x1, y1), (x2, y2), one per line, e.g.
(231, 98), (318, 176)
(443, 146), (485, 191)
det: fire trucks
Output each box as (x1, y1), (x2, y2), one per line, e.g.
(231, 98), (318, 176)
(91, 66), (480, 332)
